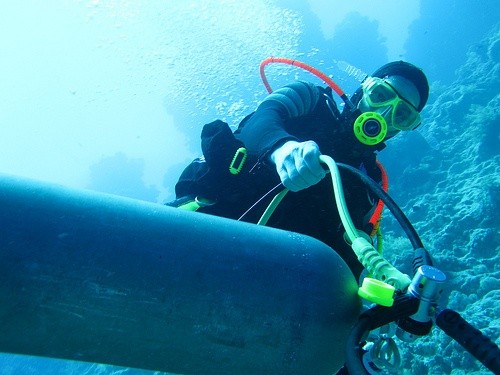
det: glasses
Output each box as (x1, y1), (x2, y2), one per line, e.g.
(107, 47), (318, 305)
(363, 76), (422, 132)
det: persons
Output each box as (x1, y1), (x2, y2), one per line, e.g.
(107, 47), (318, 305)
(165, 60), (429, 281)
